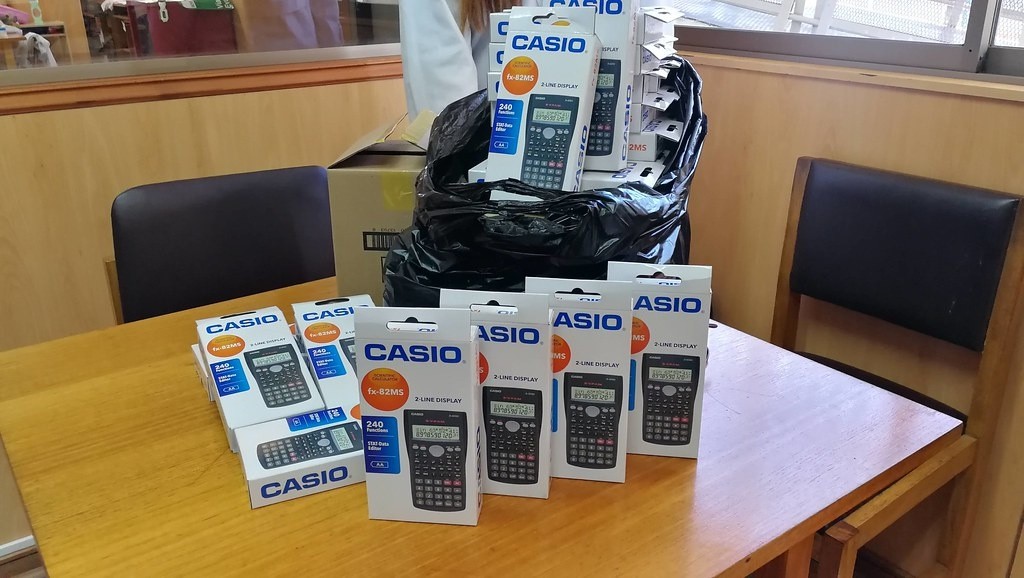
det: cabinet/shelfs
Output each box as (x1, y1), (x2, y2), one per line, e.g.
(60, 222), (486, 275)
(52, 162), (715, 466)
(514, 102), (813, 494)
(17, 21), (73, 67)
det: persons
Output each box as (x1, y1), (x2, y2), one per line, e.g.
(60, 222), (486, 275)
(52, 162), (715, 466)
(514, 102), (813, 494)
(398, 0), (554, 124)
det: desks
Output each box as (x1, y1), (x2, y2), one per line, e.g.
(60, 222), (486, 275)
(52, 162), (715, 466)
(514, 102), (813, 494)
(0, 276), (963, 578)
(0, 32), (28, 68)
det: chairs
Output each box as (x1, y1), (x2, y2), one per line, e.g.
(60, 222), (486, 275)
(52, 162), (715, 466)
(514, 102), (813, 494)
(768, 155), (1024, 578)
(103, 165), (337, 326)
(107, 4), (141, 59)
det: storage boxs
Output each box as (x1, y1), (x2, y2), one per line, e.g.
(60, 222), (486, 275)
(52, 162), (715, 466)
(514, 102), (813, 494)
(144, 1), (237, 55)
(0, 4), (29, 24)
(187, 0), (716, 527)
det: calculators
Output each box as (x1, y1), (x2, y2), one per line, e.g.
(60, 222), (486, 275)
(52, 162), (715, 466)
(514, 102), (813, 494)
(242, 343), (313, 410)
(584, 58), (622, 156)
(482, 382), (544, 484)
(254, 420), (365, 470)
(519, 93), (580, 191)
(641, 352), (700, 446)
(564, 370), (623, 469)
(339, 336), (358, 380)
(402, 408), (470, 513)
